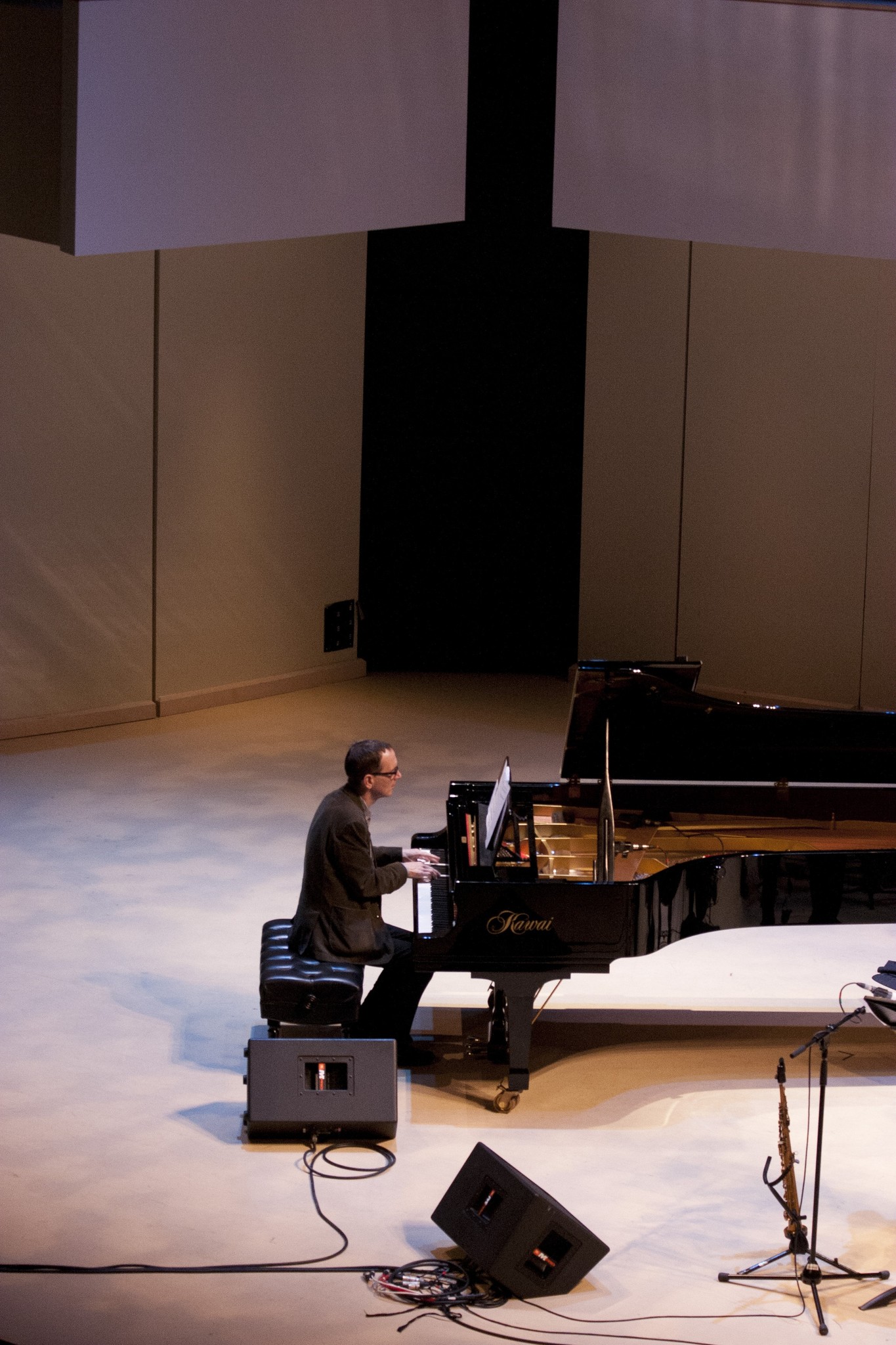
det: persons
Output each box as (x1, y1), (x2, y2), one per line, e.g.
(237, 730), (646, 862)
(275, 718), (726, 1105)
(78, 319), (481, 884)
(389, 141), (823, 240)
(287, 740), (441, 1070)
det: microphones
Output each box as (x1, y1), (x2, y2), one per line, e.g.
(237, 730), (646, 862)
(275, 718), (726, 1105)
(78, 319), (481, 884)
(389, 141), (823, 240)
(614, 842), (657, 852)
(855, 983), (896, 1000)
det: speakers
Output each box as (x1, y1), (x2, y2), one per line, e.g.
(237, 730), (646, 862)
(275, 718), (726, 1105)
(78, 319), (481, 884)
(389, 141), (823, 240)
(430, 1141), (610, 1301)
(246, 1037), (398, 1140)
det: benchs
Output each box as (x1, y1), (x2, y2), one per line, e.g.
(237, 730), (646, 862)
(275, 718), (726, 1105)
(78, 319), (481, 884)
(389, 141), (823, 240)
(259, 919), (364, 1038)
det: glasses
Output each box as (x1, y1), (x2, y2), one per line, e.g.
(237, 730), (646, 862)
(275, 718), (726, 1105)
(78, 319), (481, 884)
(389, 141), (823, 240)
(366, 766), (400, 781)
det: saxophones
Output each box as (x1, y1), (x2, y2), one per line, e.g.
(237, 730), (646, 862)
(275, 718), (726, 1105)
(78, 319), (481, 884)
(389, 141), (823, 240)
(774, 1056), (809, 1256)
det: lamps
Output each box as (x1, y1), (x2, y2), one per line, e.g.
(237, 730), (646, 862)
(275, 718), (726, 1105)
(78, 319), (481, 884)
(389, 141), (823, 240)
(718, 959), (896, 1335)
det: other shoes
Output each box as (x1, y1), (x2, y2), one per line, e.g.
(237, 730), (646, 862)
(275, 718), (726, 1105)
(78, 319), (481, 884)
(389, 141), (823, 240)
(396, 1045), (436, 1067)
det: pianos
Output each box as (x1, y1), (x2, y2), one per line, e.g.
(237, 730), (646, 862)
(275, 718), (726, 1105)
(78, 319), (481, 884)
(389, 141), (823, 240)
(411, 654), (896, 1114)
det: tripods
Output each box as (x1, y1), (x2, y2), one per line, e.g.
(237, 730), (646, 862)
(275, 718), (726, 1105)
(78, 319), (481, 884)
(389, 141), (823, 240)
(717, 1001), (889, 1335)
